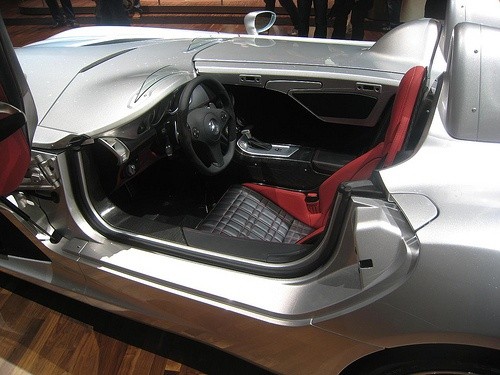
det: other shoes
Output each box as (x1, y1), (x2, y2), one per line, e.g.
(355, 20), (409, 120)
(288, 27), (299, 35)
(55, 18), (66, 27)
(67, 18), (80, 26)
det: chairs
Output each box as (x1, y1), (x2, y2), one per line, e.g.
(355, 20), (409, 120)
(195, 66), (427, 245)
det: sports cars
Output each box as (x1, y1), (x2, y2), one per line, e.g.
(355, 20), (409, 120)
(0, 0), (500, 375)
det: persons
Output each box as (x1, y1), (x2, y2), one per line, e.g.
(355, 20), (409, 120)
(44, 0), (79, 27)
(128, 0), (140, 9)
(262, 0), (299, 36)
(330, 0), (367, 40)
(95, 0), (131, 26)
(296, 0), (328, 38)
